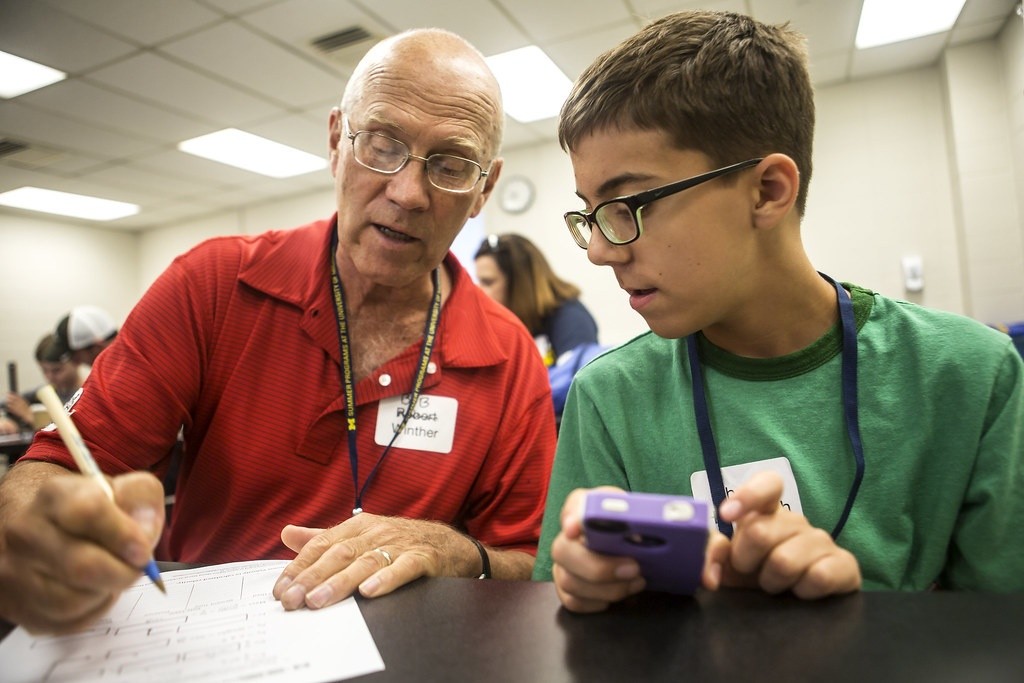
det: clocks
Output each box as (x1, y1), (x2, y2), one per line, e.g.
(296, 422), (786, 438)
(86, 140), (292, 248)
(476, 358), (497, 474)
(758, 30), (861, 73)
(496, 174), (536, 214)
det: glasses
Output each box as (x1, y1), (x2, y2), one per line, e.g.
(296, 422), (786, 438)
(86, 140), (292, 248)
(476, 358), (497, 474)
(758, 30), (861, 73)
(343, 113), (488, 192)
(564, 157), (765, 249)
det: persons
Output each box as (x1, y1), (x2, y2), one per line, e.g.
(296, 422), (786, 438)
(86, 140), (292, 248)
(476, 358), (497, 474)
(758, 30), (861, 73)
(473, 233), (599, 369)
(531, 11), (1024, 615)
(0, 26), (558, 634)
(0, 305), (120, 436)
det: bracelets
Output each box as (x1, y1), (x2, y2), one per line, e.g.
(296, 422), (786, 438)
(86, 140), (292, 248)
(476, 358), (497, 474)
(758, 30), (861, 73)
(462, 534), (492, 579)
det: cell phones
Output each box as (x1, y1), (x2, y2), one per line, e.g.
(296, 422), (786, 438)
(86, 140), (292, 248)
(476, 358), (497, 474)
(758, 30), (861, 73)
(583, 494), (710, 593)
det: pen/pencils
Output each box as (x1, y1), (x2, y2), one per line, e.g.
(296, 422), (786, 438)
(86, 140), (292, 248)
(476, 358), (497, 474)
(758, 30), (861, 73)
(36, 382), (168, 596)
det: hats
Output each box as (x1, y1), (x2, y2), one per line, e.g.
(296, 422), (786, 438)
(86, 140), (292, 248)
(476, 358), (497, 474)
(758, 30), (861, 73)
(41, 305), (116, 362)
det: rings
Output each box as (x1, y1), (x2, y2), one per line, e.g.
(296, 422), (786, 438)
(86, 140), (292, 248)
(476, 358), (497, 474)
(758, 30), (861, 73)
(375, 548), (392, 565)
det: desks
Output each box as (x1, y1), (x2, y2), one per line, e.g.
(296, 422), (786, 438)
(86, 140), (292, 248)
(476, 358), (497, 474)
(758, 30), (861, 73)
(0, 564), (1024, 683)
